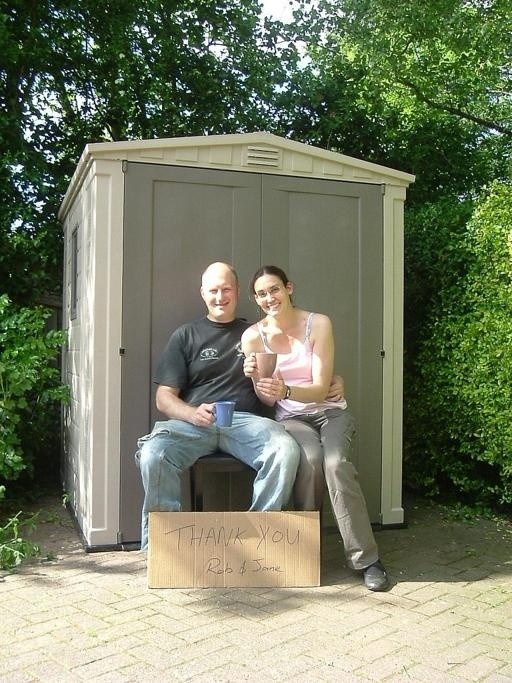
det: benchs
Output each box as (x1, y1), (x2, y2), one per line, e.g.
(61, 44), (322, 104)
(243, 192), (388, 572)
(189, 452), (256, 512)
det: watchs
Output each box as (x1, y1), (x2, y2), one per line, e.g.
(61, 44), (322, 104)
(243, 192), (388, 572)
(284, 383), (292, 403)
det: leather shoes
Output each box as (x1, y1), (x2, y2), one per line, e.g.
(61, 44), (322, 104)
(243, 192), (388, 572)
(362, 566), (389, 592)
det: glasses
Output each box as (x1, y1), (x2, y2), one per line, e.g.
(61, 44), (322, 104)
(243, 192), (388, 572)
(257, 284), (285, 298)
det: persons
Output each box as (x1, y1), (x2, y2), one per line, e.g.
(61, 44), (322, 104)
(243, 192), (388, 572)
(135, 260), (345, 553)
(240, 265), (388, 592)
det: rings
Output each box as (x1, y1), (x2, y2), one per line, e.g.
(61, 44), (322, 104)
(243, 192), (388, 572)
(338, 394), (342, 398)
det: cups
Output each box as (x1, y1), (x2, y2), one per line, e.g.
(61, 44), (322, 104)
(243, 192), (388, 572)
(254, 352), (278, 379)
(211, 401), (236, 428)
